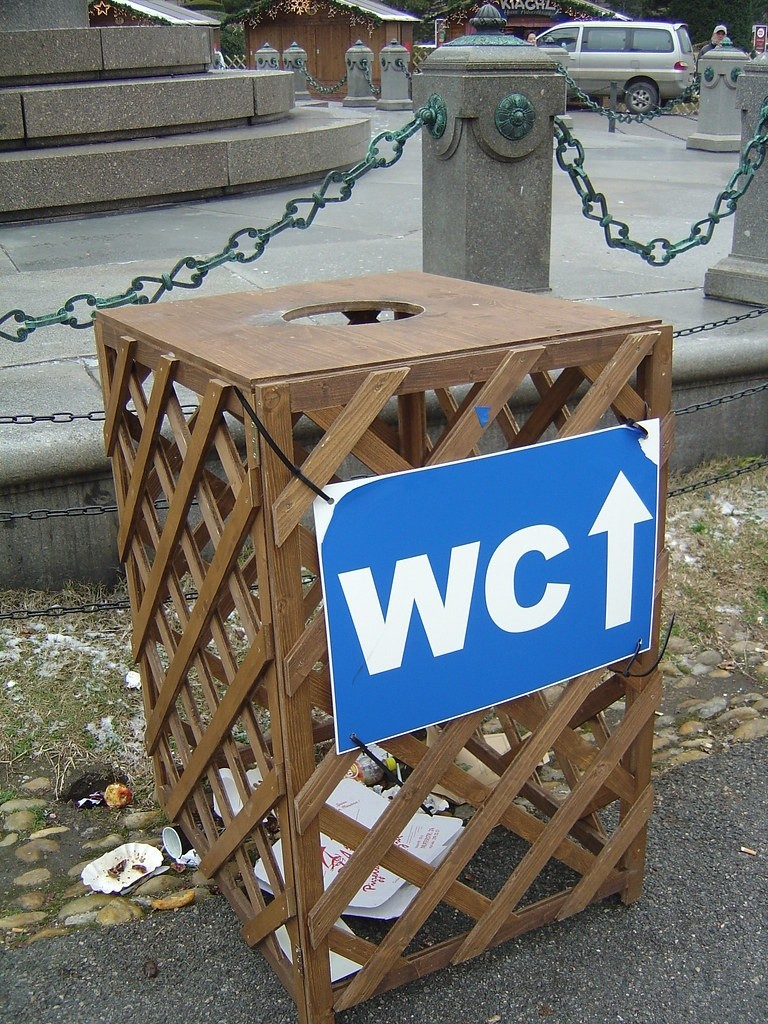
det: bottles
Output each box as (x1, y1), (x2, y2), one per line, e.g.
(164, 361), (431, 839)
(343, 757), (397, 784)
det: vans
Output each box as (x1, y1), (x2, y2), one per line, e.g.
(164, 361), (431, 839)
(538, 18), (697, 115)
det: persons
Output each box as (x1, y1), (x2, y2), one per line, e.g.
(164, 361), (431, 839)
(696, 25), (728, 71)
(523, 29), (537, 46)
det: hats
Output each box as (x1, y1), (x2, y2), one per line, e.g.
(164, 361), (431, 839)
(713, 25), (727, 34)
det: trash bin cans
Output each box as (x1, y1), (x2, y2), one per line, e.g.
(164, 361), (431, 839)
(93, 271), (673, 1024)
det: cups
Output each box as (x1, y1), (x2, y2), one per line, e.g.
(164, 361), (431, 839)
(162, 825), (188, 858)
